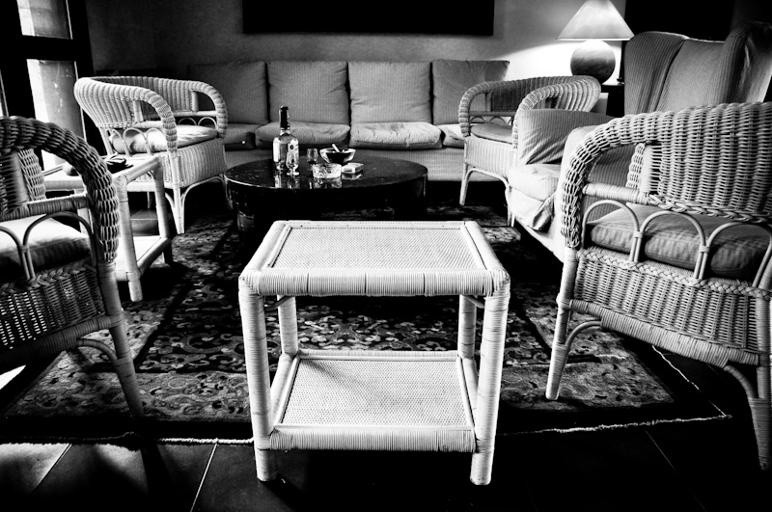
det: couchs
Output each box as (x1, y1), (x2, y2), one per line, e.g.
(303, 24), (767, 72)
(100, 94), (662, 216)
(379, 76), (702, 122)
(114, 59), (517, 185)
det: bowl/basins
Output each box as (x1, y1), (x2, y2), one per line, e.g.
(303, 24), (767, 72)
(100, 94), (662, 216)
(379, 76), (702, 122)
(320, 147), (355, 165)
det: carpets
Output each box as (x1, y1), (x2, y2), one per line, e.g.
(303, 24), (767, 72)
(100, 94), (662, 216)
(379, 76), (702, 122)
(0, 202), (729, 445)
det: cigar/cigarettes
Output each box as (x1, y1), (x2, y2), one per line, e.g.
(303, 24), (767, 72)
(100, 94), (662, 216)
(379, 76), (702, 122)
(331, 144), (340, 153)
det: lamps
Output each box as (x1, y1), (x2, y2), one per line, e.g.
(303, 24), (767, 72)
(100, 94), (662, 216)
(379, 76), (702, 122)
(557, 1), (634, 85)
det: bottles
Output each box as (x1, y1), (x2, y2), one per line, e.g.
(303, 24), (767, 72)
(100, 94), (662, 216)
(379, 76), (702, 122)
(273, 174), (287, 190)
(273, 105), (299, 173)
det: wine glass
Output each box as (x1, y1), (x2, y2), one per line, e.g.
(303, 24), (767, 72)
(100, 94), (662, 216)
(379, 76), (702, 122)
(286, 147), (318, 177)
(287, 175), (320, 190)
